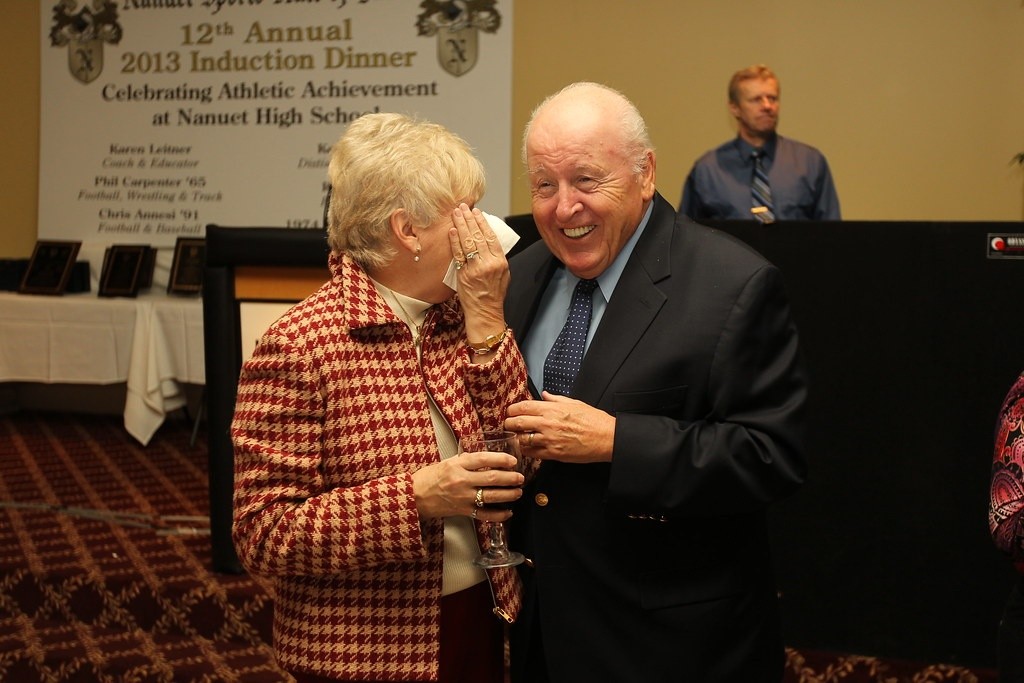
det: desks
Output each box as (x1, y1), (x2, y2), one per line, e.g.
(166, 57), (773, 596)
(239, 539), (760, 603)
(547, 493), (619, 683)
(0, 292), (304, 444)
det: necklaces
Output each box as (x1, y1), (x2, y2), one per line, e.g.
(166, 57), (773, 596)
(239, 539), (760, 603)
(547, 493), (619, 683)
(390, 290), (423, 345)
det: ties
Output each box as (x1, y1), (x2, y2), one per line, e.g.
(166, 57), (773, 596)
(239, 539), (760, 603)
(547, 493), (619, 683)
(748, 150), (774, 215)
(542, 277), (599, 401)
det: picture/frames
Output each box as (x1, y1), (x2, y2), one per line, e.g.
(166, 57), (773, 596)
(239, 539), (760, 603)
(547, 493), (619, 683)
(98, 244), (146, 297)
(19, 240), (82, 296)
(167, 238), (206, 295)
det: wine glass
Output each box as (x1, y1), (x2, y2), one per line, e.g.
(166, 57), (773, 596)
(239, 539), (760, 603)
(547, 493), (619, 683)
(458, 430), (524, 569)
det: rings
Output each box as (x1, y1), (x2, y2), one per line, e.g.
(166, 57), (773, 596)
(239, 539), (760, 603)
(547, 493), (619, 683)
(475, 489), (484, 507)
(530, 432), (535, 446)
(472, 509), (478, 518)
(454, 261), (466, 269)
(465, 250), (478, 259)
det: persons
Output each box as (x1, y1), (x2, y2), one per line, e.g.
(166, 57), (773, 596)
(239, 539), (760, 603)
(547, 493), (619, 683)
(503, 82), (810, 683)
(230, 113), (542, 683)
(988, 372), (1024, 682)
(678, 65), (844, 220)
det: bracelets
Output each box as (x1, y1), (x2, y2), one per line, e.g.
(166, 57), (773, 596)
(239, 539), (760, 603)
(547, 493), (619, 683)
(466, 323), (508, 354)
